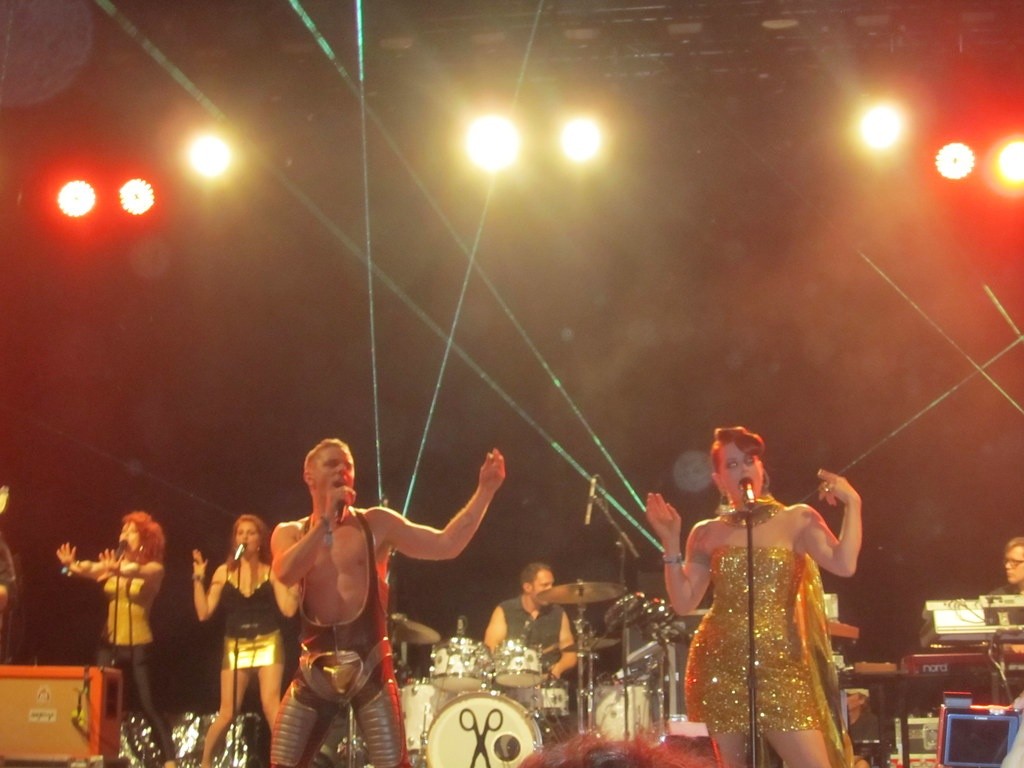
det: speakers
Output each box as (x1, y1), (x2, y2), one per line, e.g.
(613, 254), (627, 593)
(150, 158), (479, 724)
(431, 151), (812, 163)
(0, 664), (123, 761)
(936, 706), (1022, 768)
(627, 555), (714, 724)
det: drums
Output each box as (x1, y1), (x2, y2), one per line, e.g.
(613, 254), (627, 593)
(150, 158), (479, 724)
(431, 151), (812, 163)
(426, 691), (544, 768)
(396, 675), (438, 767)
(494, 637), (544, 689)
(431, 635), (494, 694)
(594, 666), (668, 751)
(533, 680), (572, 715)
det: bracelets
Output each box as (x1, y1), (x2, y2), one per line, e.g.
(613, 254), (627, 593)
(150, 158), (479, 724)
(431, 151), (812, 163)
(193, 575), (203, 580)
(320, 516), (330, 528)
(663, 555), (682, 564)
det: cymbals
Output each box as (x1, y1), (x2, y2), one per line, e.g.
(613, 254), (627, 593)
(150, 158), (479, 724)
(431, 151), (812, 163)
(387, 617), (442, 646)
(536, 582), (628, 605)
(563, 638), (621, 653)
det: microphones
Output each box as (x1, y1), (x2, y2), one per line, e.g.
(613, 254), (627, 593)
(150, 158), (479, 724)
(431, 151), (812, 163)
(738, 477), (756, 510)
(71, 709), (85, 726)
(458, 616), (465, 636)
(334, 500), (348, 524)
(114, 539), (128, 562)
(585, 477), (596, 525)
(233, 544), (246, 559)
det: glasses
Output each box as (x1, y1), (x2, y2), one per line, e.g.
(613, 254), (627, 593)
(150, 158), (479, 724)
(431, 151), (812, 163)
(1002, 558), (1024, 568)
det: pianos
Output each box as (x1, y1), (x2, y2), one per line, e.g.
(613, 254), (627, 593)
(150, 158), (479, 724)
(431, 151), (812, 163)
(900, 649), (1024, 704)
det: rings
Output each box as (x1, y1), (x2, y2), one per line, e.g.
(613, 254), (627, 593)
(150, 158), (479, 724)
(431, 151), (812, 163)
(824, 488), (830, 491)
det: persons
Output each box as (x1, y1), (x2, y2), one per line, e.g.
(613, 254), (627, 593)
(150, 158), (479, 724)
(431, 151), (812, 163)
(57, 512), (178, 768)
(192, 514), (302, 768)
(647, 428), (865, 768)
(846, 689), (878, 741)
(270, 439), (505, 767)
(0, 539), (17, 610)
(989, 535), (1024, 593)
(485, 560), (578, 679)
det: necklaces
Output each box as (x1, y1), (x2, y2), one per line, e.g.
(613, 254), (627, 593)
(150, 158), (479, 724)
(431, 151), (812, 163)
(721, 496), (780, 528)
